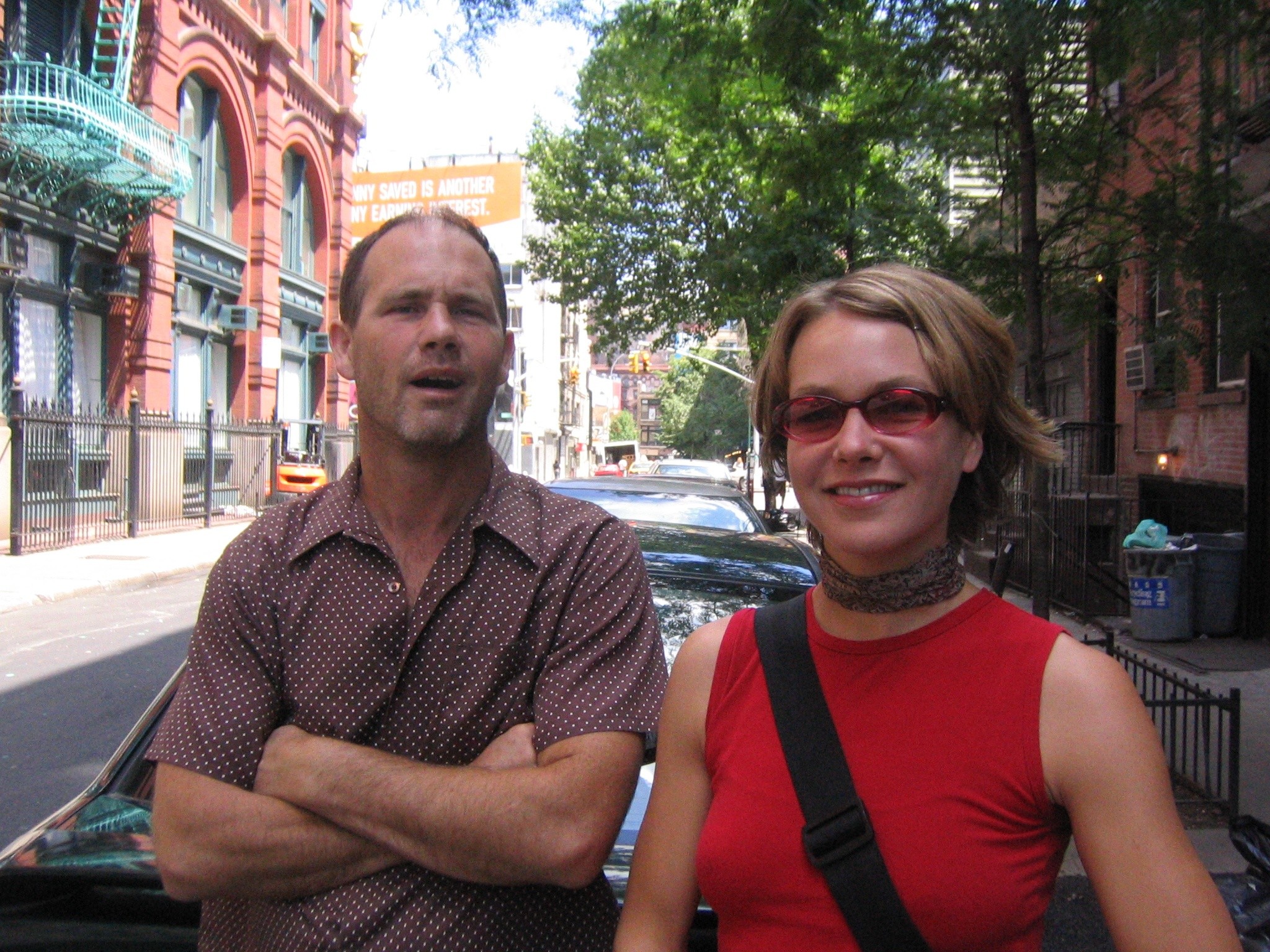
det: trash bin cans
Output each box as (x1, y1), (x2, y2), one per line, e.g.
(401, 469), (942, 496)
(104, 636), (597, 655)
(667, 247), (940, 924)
(278, 463), (328, 504)
(1168, 533), (1245, 637)
(1128, 552), (1191, 642)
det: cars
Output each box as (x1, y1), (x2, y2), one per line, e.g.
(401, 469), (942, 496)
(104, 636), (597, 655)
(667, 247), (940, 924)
(645, 458), (735, 482)
(592, 464), (624, 478)
(537, 478), (771, 535)
(2, 513), (825, 952)
(627, 460), (659, 475)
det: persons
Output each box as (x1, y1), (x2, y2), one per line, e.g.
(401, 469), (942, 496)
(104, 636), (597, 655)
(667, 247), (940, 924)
(614, 263), (1244, 952)
(144, 207), (669, 951)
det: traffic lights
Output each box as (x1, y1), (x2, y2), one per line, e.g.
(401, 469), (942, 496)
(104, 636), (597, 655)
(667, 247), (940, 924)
(628, 354), (638, 374)
(523, 435), (533, 445)
(642, 352), (651, 373)
(570, 368), (579, 385)
(523, 392), (532, 409)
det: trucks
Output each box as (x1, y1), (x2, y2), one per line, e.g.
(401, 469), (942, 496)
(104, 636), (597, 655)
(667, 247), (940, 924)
(602, 440), (640, 472)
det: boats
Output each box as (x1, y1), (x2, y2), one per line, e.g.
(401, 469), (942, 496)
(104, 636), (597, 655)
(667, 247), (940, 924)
(278, 448), (328, 495)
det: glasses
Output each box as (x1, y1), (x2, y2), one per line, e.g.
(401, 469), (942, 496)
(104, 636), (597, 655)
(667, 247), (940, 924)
(770, 386), (954, 443)
